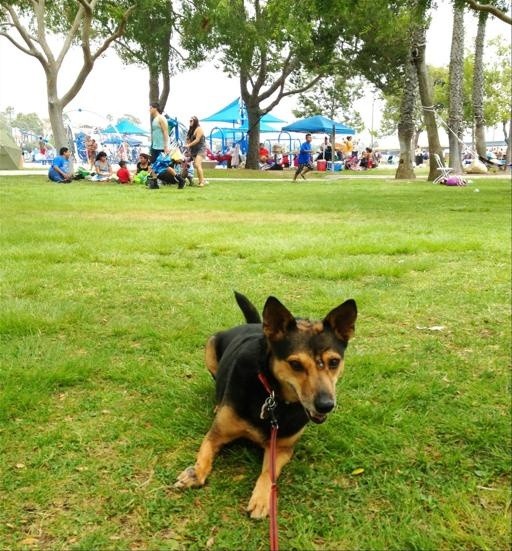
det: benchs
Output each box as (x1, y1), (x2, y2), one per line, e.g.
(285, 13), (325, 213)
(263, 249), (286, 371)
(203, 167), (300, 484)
(38, 159), (54, 165)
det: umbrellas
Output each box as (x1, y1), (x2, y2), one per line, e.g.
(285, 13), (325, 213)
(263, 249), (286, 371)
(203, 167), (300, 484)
(280, 112), (356, 159)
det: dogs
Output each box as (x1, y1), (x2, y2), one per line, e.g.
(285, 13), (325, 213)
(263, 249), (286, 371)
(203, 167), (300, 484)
(173, 288), (358, 523)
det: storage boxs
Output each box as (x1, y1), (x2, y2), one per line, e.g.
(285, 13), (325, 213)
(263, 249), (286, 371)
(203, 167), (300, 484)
(316, 159), (344, 171)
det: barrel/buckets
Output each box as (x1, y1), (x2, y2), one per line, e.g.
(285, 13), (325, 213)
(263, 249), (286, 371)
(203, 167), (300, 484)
(317, 159), (327, 171)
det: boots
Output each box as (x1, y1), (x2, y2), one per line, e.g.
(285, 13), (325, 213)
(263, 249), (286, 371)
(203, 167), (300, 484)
(146, 178), (159, 189)
(175, 173), (193, 189)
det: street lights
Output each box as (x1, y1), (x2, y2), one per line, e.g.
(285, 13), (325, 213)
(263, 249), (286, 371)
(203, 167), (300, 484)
(6, 105), (16, 136)
(370, 95), (377, 147)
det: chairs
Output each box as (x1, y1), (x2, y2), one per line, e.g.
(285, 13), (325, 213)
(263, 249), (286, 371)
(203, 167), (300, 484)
(432, 154), (453, 184)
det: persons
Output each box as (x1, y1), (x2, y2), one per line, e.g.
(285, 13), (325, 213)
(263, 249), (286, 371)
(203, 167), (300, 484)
(115, 160), (134, 185)
(146, 102), (187, 191)
(185, 114), (208, 188)
(38, 136), (46, 155)
(131, 152), (152, 186)
(259, 142), (269, 156)
(47, 146), (73, 184)
(84, 136), (142, 173)
(291, 132), (316, 182)
(230, 142), (241, 170)
(90, 151), (115, 184)
(314, 135), (382, 171)
(183, 150), (197, 187)
